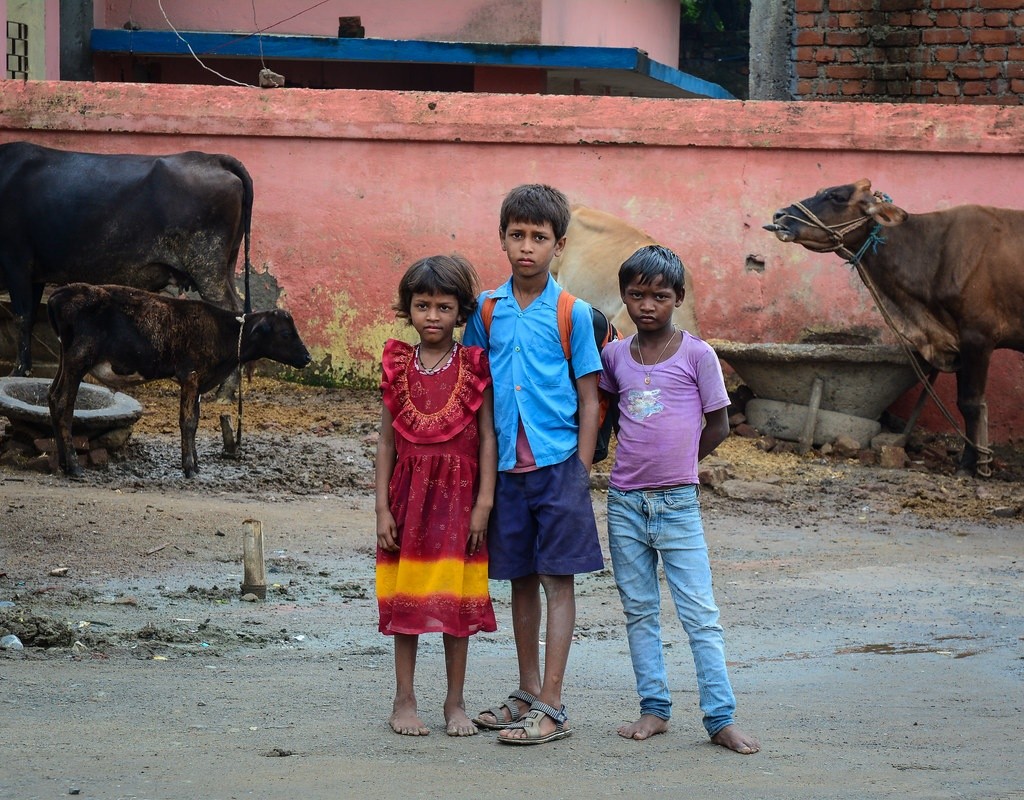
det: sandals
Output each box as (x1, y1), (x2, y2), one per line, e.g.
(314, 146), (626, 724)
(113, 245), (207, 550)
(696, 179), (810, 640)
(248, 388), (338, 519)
(471, 691), (537, 728)
(497, 700), (574, 745)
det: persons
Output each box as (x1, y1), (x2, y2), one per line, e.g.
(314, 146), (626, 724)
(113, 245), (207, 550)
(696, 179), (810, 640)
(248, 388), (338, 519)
(461, 183), (603, 744)
(374, 255), (498, 737)
(599, 245), (760, 754)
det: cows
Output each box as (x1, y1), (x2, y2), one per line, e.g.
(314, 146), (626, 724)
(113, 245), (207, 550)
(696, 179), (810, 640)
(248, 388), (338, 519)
(0, 142), (311, 480)
(761, 179), (1024, 480)
(545, 206), (698, 341)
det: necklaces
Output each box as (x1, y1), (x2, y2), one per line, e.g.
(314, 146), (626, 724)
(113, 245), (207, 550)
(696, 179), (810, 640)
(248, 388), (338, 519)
(418, 341), (456, 376)
(637, 329), (678, 385)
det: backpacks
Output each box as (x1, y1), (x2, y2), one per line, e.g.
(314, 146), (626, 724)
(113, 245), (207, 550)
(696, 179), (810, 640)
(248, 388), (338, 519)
(481, 285), (625, 464)
